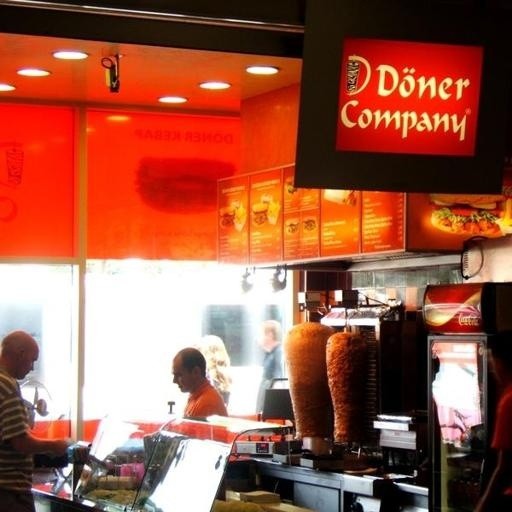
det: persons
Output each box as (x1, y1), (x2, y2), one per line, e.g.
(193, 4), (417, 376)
(0, 330), (69, 512)
(255, 320), (285, 415)
(193, 335), (233, 405)
(473, 331), (512, 512)
(171, 347), (229, 418)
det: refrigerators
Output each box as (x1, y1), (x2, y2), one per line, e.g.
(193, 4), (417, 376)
(422, 282), (511, 512)
